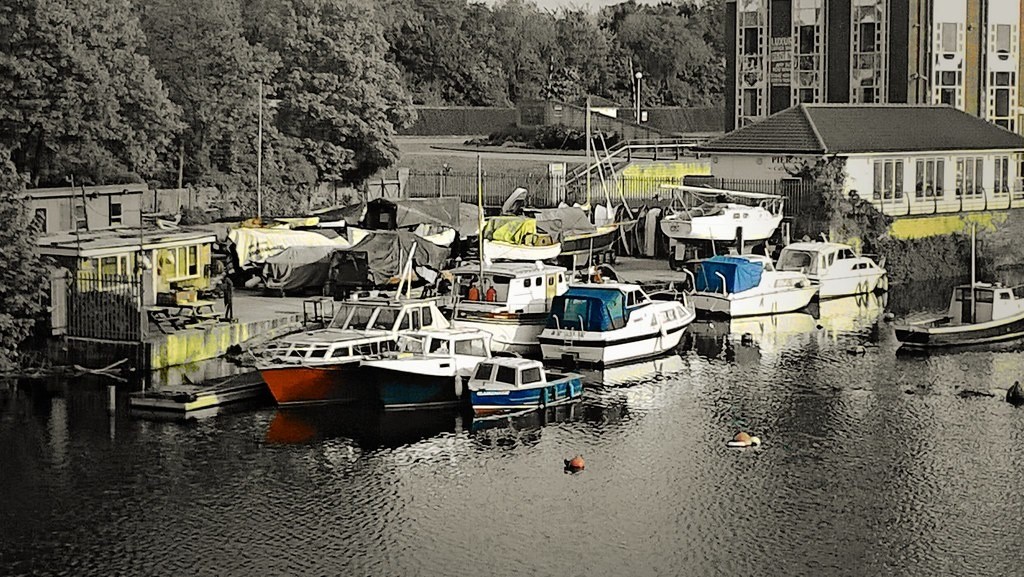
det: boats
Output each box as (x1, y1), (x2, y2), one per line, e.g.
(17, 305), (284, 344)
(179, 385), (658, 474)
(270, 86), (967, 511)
(775, 231), (890, 301)
(467, 356), (586, 421)
(685, 310), (818, 340)
(574, 354), (685, 387)
(682, 254), (819, 319)
(535, 278), (697, 367)
(811, 292), (879, 315)
(246, 96), (620, 414)
(658, 182), (786, 243)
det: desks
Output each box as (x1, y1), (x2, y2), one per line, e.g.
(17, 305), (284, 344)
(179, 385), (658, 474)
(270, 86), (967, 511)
(147, 306), (181, 334)
(303, 296), (335, 325)
(177, 302), (221, 323)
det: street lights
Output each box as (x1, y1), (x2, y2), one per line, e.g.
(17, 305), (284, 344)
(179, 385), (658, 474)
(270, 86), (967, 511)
(635, 71), (643, 125)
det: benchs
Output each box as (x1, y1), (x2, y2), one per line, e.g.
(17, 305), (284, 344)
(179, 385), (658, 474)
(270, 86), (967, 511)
(155, 317), (180, 322)
(195, 311), (224, 319)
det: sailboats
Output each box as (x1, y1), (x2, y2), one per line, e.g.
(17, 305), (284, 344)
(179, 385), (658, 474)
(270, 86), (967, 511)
(893, 222), (1023, 350)
